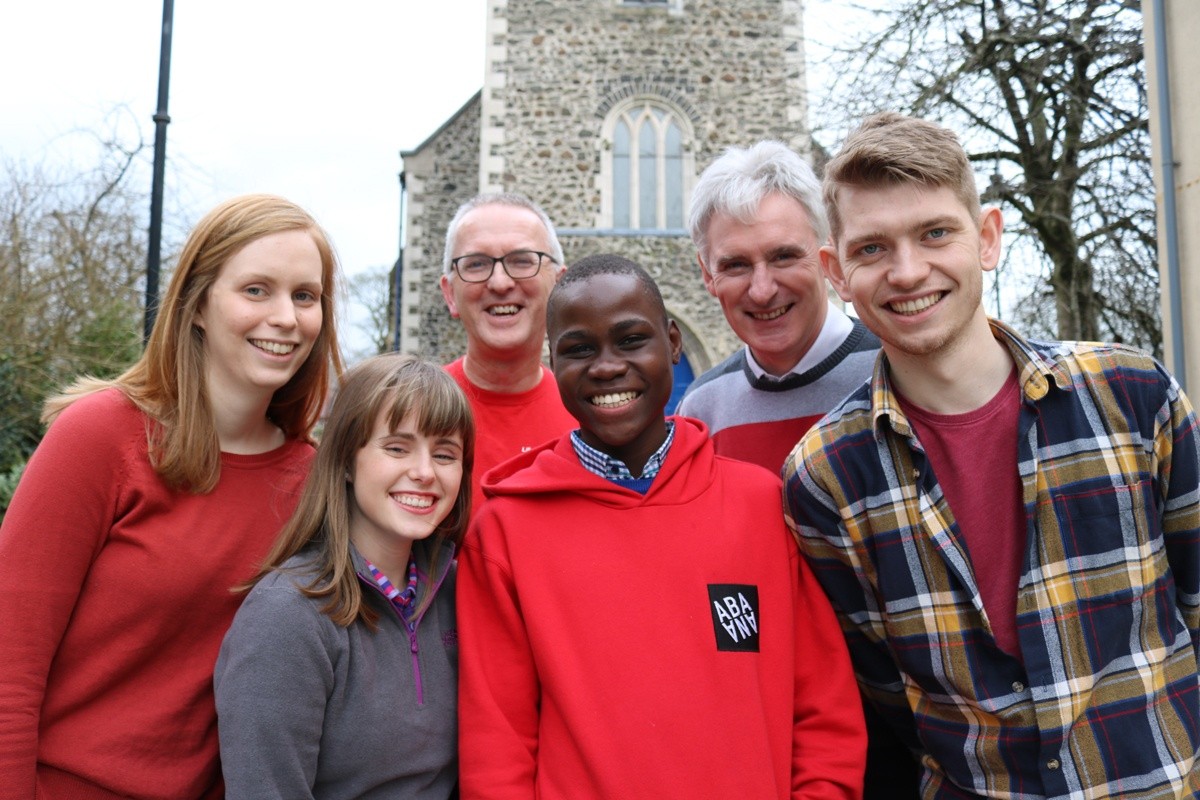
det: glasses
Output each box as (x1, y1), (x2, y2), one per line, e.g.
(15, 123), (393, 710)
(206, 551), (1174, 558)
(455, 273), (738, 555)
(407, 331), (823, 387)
(451, 250), (560, 283)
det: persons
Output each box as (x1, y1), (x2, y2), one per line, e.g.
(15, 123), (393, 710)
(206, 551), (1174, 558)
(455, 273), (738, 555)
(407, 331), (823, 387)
(440, 193), (580, 526)
(674, 142), (883, 480)
(780, 114), (1200, 799)
(1, 195), (346, 800)
(213, 353), (475, 800)
(456, 254), (866, 799)
